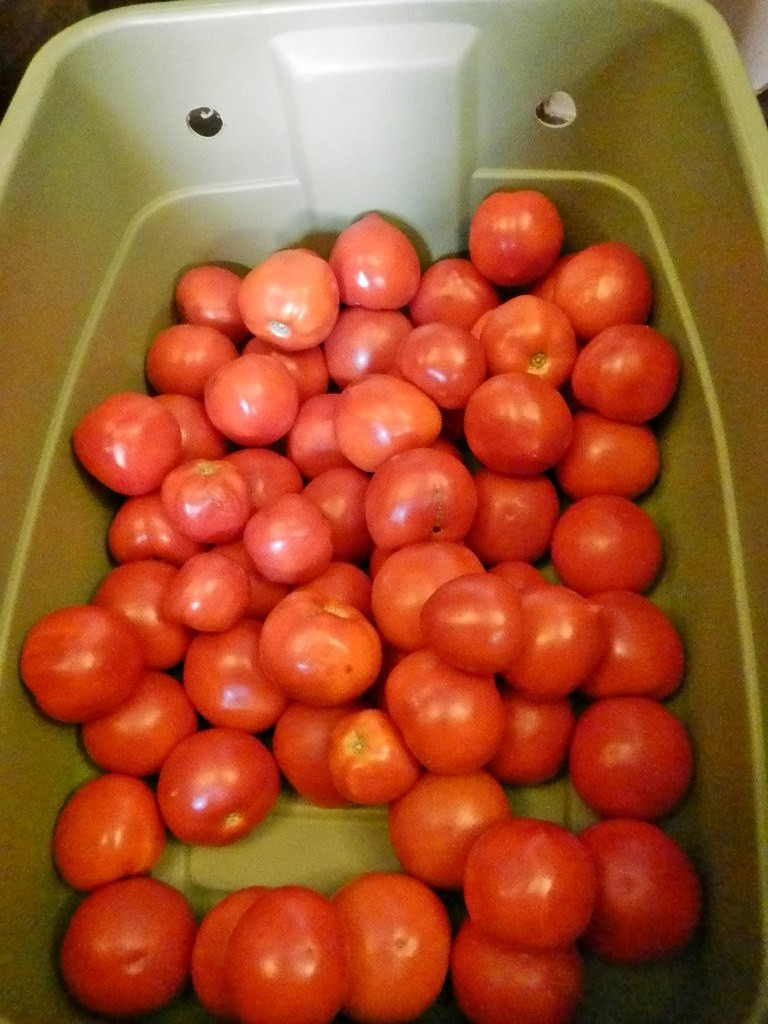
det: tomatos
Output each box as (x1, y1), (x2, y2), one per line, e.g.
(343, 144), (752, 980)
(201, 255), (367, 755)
(20, 189), (697, 1024)
